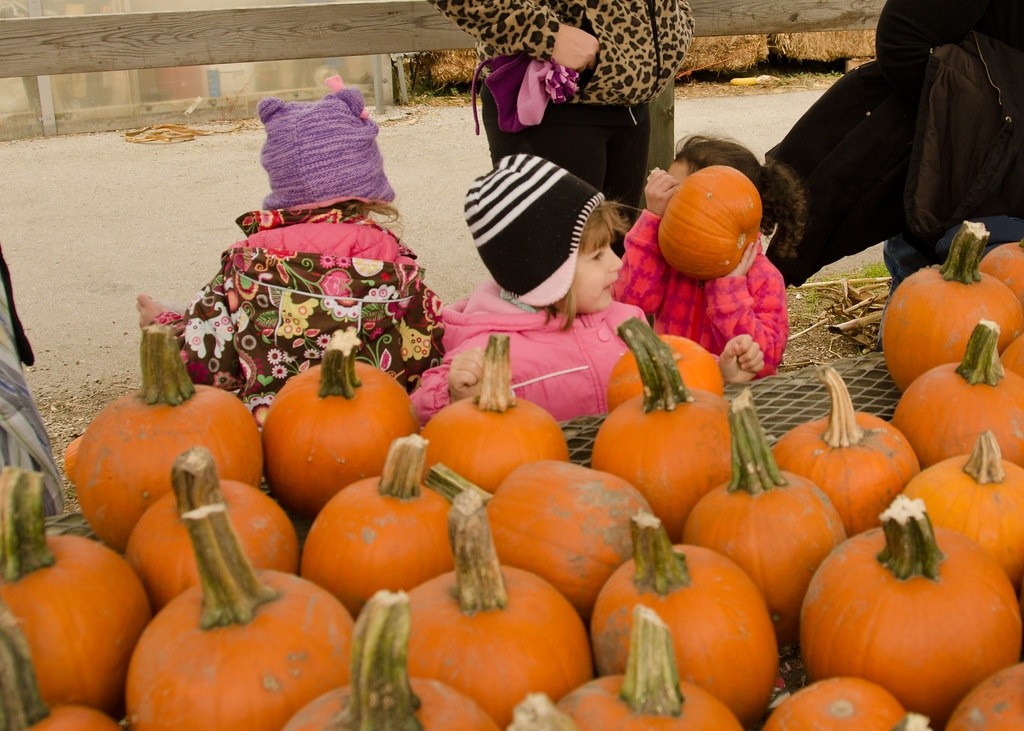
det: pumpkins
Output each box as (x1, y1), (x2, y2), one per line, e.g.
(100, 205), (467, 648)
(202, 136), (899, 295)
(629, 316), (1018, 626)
(657, 165), (762, 280)
(0, 221), (1024, 731)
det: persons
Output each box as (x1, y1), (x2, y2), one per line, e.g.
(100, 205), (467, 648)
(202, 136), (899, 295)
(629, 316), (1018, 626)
(136, 89), (444, 431)
(767, 0), (1024, 296)
(408, 154), (763, 426)
(431, 0), (698, 256)
(611, 137), (806, 378)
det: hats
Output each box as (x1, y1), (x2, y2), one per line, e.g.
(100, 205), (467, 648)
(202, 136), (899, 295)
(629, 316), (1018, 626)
(256, 73), (396, 211)
(462, 153), (605, 307)
(472, 50), (580, 136)
